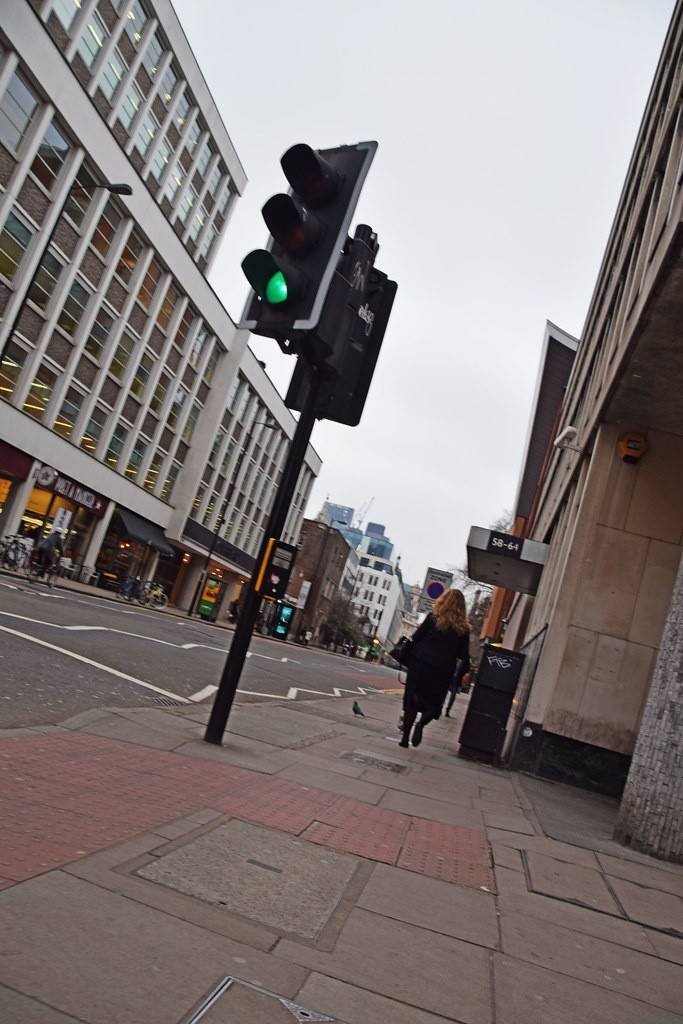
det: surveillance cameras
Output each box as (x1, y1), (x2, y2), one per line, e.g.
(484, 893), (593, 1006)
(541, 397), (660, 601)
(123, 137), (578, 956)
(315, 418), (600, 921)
(554, 426), (577, 450)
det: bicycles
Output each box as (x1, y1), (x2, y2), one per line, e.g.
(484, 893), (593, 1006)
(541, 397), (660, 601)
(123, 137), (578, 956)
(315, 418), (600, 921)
(0, 533), (64, 589)
(117, 574), (170, 610)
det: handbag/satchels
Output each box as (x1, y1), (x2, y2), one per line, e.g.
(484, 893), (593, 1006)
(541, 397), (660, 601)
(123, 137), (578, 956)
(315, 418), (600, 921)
(390, 636), (411, 683)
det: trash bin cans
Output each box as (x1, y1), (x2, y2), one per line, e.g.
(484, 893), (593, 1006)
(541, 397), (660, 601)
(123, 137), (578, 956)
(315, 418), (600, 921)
(294, 621), (314, 646)
(457, 642), (526, 765)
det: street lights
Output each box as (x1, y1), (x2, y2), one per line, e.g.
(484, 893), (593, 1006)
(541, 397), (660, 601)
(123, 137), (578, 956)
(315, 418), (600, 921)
(0, 182), (133, 369)
(295, 517), (347, 645)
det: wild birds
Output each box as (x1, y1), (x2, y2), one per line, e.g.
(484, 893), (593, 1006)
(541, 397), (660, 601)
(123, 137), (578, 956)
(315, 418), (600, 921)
(352, 700), (364, 717)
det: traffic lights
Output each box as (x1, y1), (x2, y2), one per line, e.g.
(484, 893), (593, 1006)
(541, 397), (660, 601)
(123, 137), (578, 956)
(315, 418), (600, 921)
(237, 137), (380, 332)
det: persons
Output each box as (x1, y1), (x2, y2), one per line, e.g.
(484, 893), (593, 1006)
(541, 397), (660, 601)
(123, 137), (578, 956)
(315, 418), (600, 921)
(326, 636), (334, 650)
(229, 600), (240, 624)
(295, 625), (313, 646)
(350, 643), (358, 656)
(37, 526), (64, 579)
(17, 521), (44, 546)
(399, 588), (474, 748)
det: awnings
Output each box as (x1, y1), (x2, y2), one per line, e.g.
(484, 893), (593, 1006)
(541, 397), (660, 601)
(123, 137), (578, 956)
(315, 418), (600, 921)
(115, 507), (176, 557)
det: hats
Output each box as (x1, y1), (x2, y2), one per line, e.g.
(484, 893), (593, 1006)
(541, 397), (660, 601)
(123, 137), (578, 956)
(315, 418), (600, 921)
(55, 527), (64, 534)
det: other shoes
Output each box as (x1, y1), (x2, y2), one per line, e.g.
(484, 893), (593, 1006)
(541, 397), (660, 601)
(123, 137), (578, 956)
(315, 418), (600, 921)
(399, 737), (409, 748)
(445, 710), (449, 717)
(412, 722), (423, 747)
